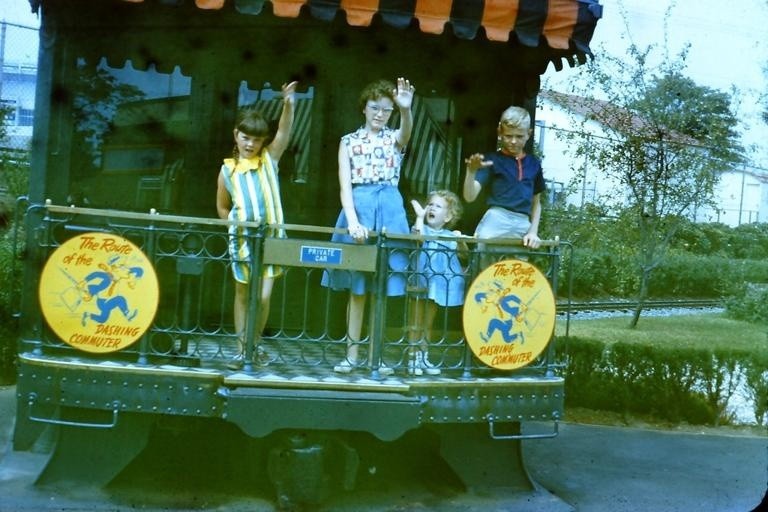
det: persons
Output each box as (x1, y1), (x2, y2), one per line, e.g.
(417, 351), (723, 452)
(463, 104), (546, 268)
(214, 80), (295, 370)
(318, 76), (415, 376)
(403, 185), (472, 375)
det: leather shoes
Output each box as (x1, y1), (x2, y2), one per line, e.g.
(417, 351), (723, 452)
(81, 312), (90, 328)
(480, 332), (490, 343)
(518, 332), (526, 346)
(127, 309), (138, 322)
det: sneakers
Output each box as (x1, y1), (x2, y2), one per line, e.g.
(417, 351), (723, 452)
(251, 342), (269, 368)
(418, 362), (442, 376)
(333, 357), (362, 373)
(405, 360), (423, 377)
(226, 349), (248, 371)
(364, 359), (395, 376)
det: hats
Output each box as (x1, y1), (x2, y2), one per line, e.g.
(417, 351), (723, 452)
(107, 254), (124, 265)
(491, 281), (503, 291)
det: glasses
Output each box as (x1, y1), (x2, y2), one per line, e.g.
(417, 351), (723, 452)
(366, 102), (393, 116)
